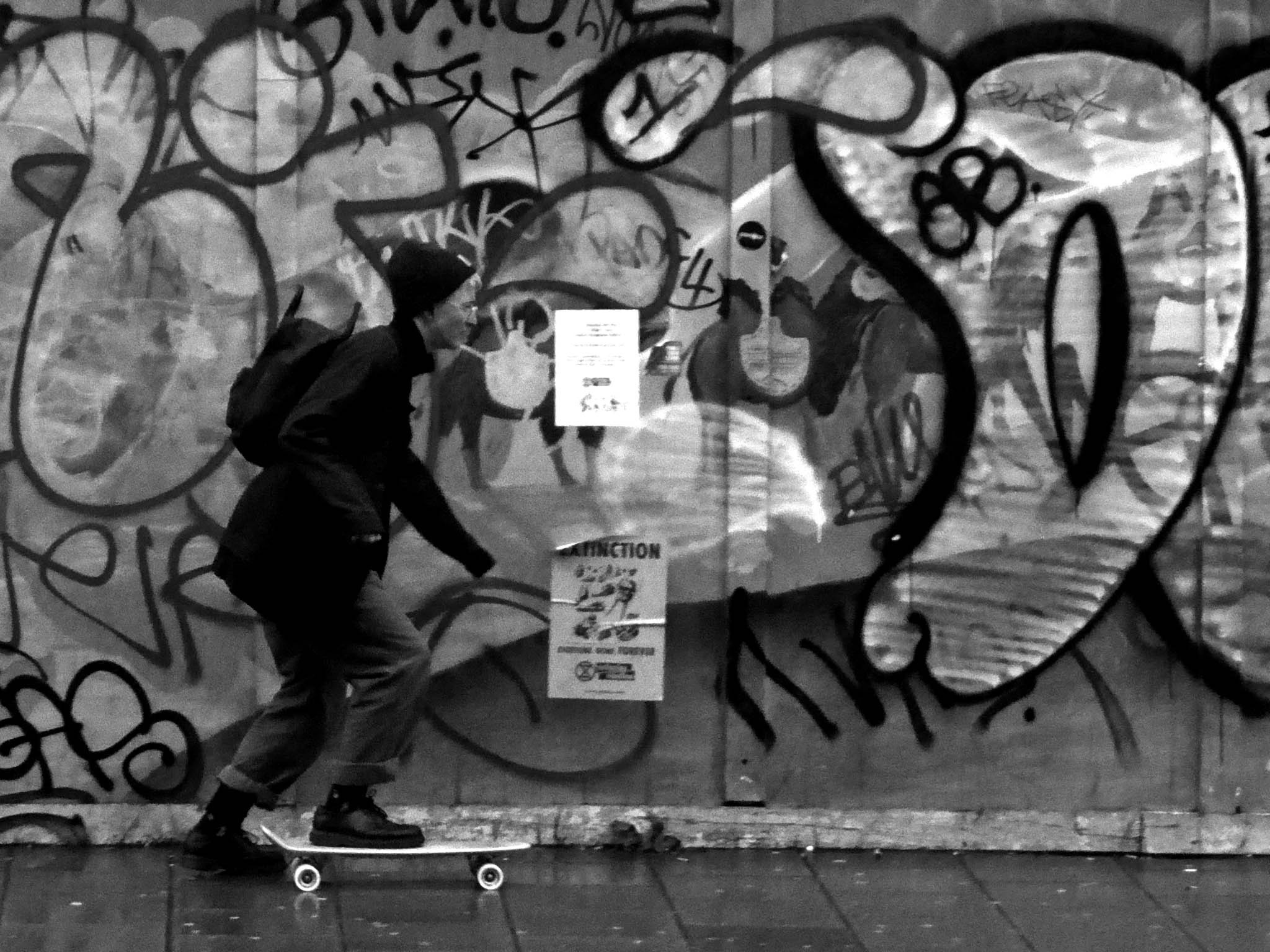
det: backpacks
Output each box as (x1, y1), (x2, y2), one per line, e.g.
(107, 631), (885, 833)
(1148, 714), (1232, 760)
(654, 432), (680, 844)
(225, 285), (365, 467)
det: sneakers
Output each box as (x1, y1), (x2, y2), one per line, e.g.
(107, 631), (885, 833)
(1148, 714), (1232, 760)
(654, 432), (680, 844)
(310, 788), (427, 847)
(183, 822), (284, 874)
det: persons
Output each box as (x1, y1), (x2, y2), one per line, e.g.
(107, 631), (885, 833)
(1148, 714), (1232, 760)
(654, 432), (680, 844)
(182, 239), (497, 877)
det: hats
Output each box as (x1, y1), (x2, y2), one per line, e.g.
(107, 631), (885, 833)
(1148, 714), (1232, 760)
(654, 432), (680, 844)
(384, 240), (478, 323)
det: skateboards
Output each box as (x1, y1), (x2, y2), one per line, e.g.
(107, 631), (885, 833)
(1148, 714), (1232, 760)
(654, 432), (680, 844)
(259, 825), (530, 891)
